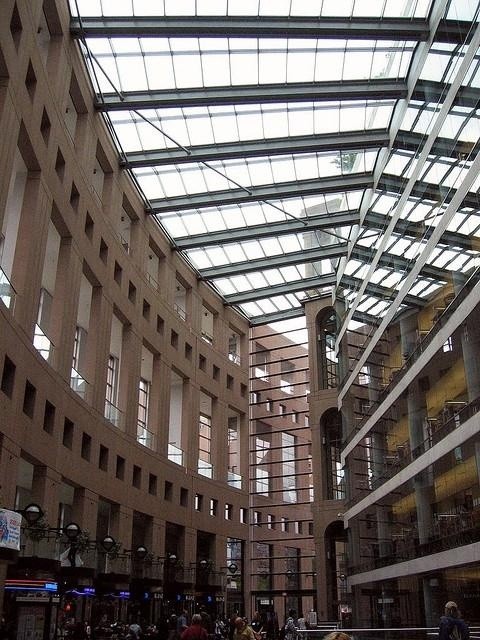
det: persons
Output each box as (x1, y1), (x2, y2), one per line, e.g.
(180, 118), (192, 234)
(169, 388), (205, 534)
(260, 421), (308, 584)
(56, 604), (322, 640)
(437, 600), (471, 640)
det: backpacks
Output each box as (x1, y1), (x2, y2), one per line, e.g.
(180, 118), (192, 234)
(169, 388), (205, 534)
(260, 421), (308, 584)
(279, 618), (291, 639)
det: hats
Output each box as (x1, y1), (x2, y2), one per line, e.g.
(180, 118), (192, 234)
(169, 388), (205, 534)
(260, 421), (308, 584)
(445, 602), (457, 608)
